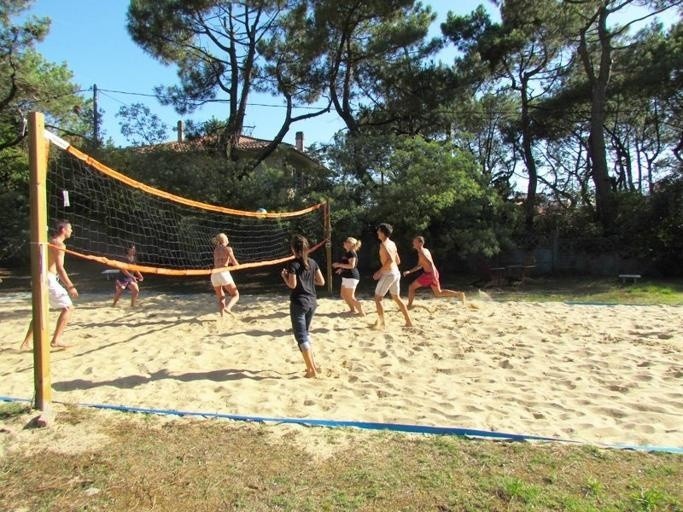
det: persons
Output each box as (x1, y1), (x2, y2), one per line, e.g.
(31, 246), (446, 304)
(330, 236), (367, 319)
(208, 231), (240, 320)
(17, 219), (80, 353)
(402, 234), (465, 309)
(366, 222), (414, 330)
(278, 233), (326, 381)
(110, 243), (143, 311)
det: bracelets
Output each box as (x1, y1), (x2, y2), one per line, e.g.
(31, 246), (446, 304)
(405, 270), (411, 274)
(67, 285), (75, 291)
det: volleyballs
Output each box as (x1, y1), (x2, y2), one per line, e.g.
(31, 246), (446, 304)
(256, 208), (267, 219)
